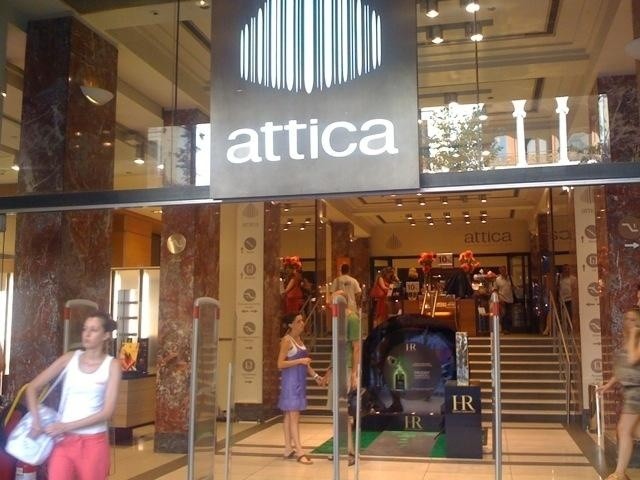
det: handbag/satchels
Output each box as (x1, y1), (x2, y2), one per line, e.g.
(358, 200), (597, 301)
(4, 403), (64, 466)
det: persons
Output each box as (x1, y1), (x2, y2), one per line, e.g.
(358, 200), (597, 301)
(321, 289), (362, 466)
(543, 311), (551, 336)
(374, 266), (396, 325)
(277, 311), (324, 464)
(281, 266), (306, 320)
(492, 265), (520, 333)
(594, 309), (640, 480)
(559, 264), (577, 334)
(26, 310), (122, 480)
(330, 264), (362, 313)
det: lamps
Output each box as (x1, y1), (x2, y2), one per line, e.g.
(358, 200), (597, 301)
(419, 0), (483, 45)
(396, 193), (489, 226)
(282, 203), (312, 231)
(80, 83), (115, 106)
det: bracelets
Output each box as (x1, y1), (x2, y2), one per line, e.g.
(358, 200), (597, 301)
(312, 374), (318, 381)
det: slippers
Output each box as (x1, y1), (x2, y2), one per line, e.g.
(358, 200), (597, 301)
(287, 450), (313, 464)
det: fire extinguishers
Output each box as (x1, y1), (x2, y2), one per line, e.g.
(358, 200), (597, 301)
(15, 461), (37, 480)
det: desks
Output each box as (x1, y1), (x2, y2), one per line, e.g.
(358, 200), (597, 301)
(108, 374), (156, 446)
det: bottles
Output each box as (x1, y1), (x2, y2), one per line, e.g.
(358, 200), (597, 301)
(393, 365), (406, 391)
